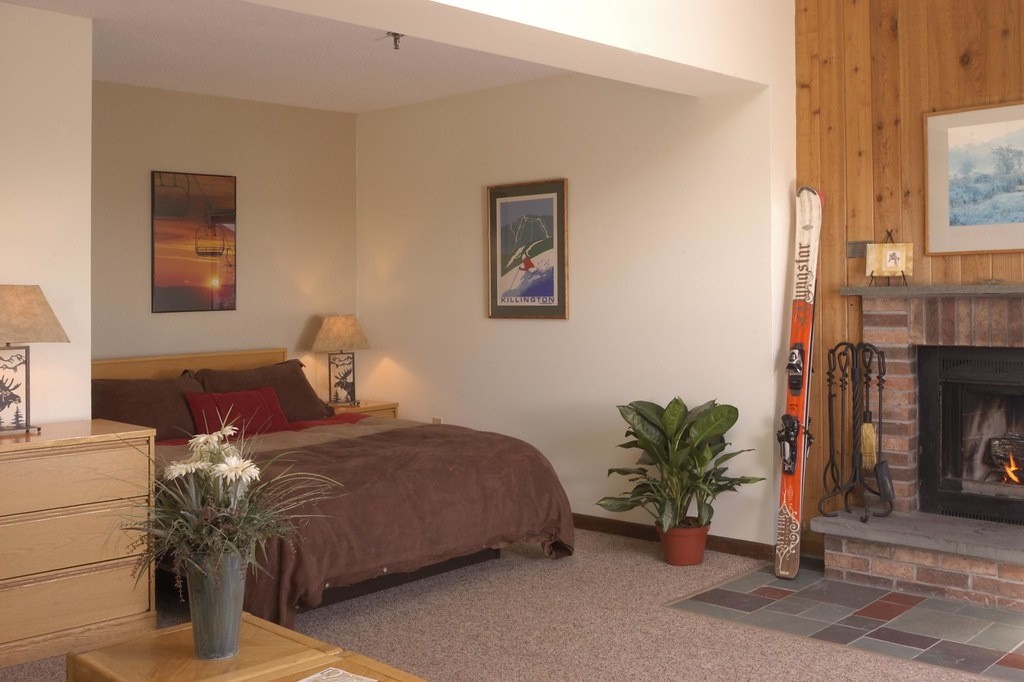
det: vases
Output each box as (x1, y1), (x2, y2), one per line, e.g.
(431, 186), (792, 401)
(185, 546), (249, 660)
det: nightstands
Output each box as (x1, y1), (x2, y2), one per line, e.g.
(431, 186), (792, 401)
(323, 399), (399, 420)
(0, 417), (159, 670)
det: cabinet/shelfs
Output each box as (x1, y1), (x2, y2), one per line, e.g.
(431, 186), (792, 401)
(66, 607), (343, 682)
(241, 650), (424, 682)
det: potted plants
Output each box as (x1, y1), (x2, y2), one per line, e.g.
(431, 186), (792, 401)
(594, 395), (768, 566)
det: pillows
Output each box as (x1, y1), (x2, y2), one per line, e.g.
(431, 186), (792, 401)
(91, 369), (205, 442)
(194, 358), (334, 423)
(184, 386), (289, 437)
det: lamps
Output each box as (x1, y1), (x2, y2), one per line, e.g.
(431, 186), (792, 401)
(0, 283), (71, 435)
(310, 314), (372, 406)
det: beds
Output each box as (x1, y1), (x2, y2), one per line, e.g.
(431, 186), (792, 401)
(91, 347), (576, 630)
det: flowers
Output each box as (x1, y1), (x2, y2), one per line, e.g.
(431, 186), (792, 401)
(106, 402), (348, 604)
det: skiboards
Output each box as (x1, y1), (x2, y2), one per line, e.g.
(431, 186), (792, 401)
(772, 184), (823, 581)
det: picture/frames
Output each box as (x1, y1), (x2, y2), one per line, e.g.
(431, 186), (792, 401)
(922, 101), (1024, 256)
(481, 177), (569, 320)
(328, 352), (356, 404)
(149, 170), (238, 313)
(0, 345), (31, 431)
